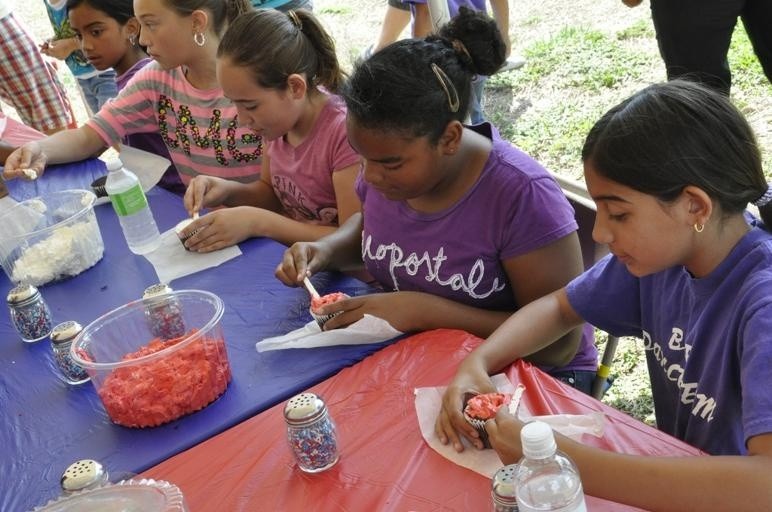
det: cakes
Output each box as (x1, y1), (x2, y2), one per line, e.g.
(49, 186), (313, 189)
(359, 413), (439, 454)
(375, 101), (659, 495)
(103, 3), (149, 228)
(464, 392), (520, 450)
(309, 292), (352, 333)
(176, 218), (210, 251)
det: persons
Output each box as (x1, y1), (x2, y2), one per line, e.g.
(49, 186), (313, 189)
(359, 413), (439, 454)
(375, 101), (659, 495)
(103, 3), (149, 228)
(349, 0), (512, 133)
(0, 6), (78, 144)
(38, 1), (120, 117)
(432, 80), (771, 512)
(619, 0), (770, 108)
(65, 0), (189, 197)
(175, 8), (379, 290)
(2, 2), (266, 216)
(64, 1), (153, 126)
(272, 3), (598, 404)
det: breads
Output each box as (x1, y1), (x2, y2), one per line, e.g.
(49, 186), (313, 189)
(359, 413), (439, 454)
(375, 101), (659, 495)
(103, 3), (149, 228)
(90, 175), (108, 198)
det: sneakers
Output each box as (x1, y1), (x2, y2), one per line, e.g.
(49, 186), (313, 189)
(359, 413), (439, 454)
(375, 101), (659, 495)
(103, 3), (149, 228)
(495, 57), (526, 72)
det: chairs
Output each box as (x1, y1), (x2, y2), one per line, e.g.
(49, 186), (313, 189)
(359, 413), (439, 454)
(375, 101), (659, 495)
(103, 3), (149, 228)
(552, 176), (618, 400)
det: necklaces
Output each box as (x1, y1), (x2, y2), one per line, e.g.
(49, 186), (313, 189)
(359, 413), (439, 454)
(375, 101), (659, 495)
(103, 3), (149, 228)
(185, 69), (188, 81)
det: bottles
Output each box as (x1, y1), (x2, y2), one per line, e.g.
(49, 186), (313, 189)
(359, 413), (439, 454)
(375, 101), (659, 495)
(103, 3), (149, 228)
(142, 283), (187, 339)
(490, 464), (530, 512)
(48, 320), (95, 385)
(7, 284), (52, 343)
(283, 392), (339, 473)
(105, 156), (161, 256)
(513, 421), (587, 512)
